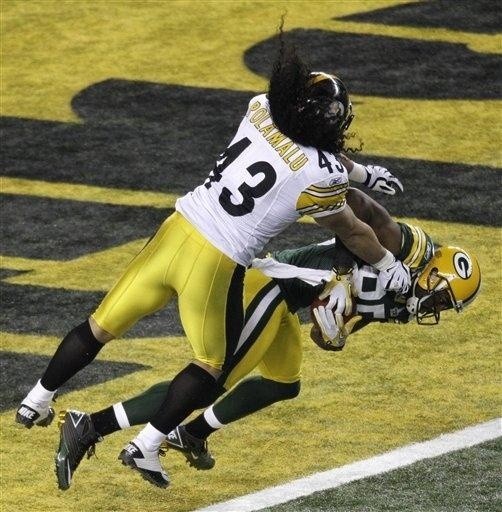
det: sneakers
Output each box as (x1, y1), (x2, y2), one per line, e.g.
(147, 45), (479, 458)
(118, 439), (168, 488)
(165, 426), (215, 470)
(54, 409), (104, 490)
(16, 398), (55, 429)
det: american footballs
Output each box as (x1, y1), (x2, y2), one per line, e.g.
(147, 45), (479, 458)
(311, 295), (356, 325)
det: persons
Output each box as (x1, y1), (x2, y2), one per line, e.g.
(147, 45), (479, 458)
(11, 7), (412, 490)
(52, 177), (483, 493)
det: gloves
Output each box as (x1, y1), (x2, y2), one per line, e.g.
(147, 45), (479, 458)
(318, 264), (358, 315)
(382, 259), (412, 294)
(311, 306), (363, 351)
(364, 164), (404, 195)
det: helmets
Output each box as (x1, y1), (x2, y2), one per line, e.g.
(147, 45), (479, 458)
(298, 72), (354, 130)
(411, 247), (481, 325)
